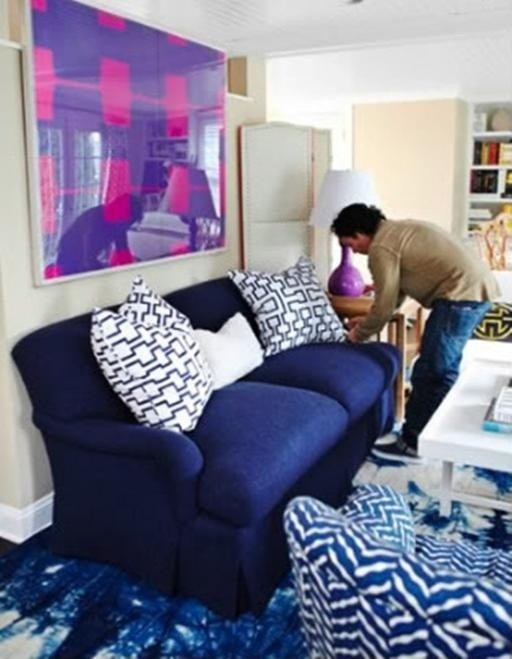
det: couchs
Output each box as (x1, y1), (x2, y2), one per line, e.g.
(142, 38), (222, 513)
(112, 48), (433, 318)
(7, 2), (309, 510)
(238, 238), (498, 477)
(10, 277), (404, 625)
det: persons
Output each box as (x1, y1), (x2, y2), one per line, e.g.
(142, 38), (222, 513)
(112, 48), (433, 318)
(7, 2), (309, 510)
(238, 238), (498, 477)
(330, 202), (502, 464)
(56, 193), (144, 276)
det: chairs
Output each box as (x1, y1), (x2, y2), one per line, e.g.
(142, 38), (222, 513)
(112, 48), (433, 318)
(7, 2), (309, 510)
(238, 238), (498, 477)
(283, 482), (511, 658)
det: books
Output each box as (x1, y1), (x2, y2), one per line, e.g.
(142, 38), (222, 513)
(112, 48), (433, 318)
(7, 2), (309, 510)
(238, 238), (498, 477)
(151, 141), (186, 160)
(467, 141), (512, 229)
(479, 375), (512, 434)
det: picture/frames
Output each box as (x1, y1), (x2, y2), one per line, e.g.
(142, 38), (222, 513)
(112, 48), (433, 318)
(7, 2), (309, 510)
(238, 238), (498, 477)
(22, 2), (228, 286)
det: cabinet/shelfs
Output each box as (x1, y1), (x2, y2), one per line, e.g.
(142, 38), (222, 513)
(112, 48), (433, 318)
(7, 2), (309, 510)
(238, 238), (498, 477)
(241, 121), (332, 274)
(464, 97), (511, 236)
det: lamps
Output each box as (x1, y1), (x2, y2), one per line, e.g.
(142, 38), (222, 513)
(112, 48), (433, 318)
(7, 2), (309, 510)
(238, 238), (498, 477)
(307, 170), (383, 297)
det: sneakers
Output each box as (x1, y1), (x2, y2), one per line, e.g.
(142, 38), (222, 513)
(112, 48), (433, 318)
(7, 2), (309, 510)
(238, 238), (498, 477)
(370, 442), (418, 464)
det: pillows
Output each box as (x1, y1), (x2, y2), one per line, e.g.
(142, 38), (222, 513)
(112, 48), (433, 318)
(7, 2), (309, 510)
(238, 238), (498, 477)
(193, 313), (264, 391)
(90, 273), (215, 433)
(225, 251), (346, 357)
